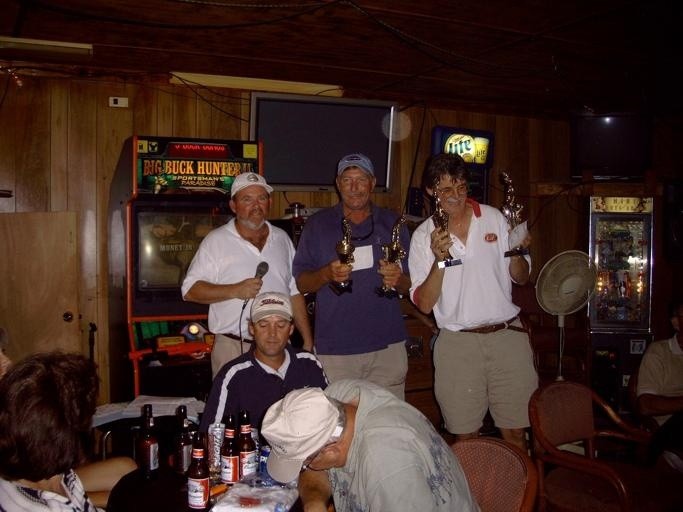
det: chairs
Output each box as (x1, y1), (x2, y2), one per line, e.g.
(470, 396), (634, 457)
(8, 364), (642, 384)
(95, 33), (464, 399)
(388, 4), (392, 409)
(629, 367), (674, 433)
(451, 437), (537, 512)
(529, 381), (681, 512)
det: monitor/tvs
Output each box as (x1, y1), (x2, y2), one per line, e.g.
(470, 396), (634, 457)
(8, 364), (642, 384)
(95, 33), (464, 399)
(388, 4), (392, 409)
(245, 89), (402, 193)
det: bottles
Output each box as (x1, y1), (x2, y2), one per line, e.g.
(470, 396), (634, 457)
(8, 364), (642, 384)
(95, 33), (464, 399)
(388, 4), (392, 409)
(239, 408), (256, 479)
(185, 432), (210, 510)
(138, 404), (159, 479)
(220, 411), (240, 485)
(172, 405), (192, 477)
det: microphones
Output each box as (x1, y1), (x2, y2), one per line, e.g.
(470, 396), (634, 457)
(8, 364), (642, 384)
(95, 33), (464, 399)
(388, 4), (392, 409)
(242, 262), (270, 306)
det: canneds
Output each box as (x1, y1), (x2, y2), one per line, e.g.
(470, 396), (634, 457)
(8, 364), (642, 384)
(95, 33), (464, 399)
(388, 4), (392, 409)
(209, 423), (225, 466)
(258, 445), (273, 485)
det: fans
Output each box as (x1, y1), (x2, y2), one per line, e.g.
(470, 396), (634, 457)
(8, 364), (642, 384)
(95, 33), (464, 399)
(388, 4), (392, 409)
(535, 251), (599, 382)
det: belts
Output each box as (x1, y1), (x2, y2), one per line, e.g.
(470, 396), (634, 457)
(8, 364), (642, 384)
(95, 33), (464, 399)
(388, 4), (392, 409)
(460, 317), (529, 335)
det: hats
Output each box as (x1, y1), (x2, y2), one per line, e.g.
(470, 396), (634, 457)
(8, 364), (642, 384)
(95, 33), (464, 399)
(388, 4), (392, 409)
(250, 292), (294, 324)
(230, 172), (274, 198)
(260, 387), (339, 483)
(338, 153), (374, 179)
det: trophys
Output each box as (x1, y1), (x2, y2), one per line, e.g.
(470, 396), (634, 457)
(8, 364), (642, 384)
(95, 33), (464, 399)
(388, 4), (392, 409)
(375, 212), (407, 301)
(500, 167), (530, 258)
(330, 217), (355, 296)
(432, 175), (462, 269)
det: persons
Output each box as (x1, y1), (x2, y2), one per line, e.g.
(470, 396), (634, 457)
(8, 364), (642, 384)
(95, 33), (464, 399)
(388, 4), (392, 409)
(73, 454), (138, 494)
(263, 379), (482, 512)
(179, 172), (313, 379)
(291, 150), (408, 409)
(198, 290), (332, 447)
(0, 352), (103, 512)
(407, 153), (539, 456)
(636, 301), (683, 442)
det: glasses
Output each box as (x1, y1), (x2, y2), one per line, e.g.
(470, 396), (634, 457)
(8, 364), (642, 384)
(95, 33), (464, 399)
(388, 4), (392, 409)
(300, 440), (337, 474)
